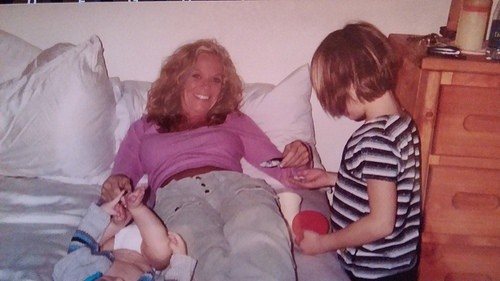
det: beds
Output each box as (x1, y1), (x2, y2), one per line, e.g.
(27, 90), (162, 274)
(0, 83), (353, 281)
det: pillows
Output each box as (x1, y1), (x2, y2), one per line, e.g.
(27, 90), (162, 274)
(239, 62), (331, 194)
(0, 30), (42, 84)
(109, 77), (153, 157)
(0, 35), (116, 185)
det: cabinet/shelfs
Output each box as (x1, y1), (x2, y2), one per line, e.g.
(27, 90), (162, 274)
(381, 34), (500, 281)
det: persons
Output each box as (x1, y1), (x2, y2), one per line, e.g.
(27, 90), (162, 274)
(52, 187), (197, 281)
(287, 21), (422, 281)
(100, 38), (314, 281)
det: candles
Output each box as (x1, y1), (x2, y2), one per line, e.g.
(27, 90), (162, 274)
(455, 0), (492, 50)
